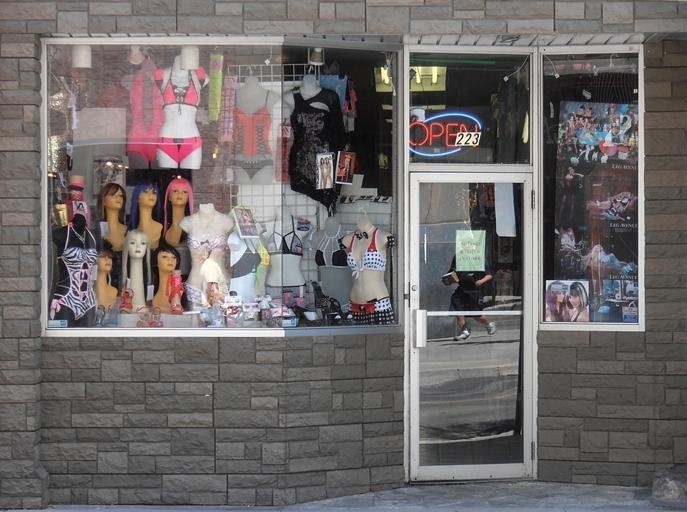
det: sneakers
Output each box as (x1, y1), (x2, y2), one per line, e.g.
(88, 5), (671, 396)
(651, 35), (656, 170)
(454, 329), (471, 341)
(485, 322), (496, 335)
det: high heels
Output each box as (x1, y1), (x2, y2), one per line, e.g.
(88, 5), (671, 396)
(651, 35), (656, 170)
(293, 282), (353, 326)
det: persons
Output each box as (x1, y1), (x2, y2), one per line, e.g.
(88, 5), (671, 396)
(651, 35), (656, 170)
(442, 253), (496, 341)
(319, 157), (333, 189)
(232, 77), (280, 184)
(228, 203), (396, 327)
(49, 56), (74, 176)
(545, 281), (589, 322)
(343, 153), (352, 171)
(283, 74), (347, 210)
(100, 55), (210, 169)
(50, 179), (236, 326)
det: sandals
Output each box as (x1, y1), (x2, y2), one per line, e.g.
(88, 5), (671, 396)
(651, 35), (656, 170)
(92, 288), (164, 328)
(169, 277), (297, 328)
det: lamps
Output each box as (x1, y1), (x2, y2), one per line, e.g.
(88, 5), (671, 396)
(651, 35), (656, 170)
(127, 46), (146, 65)
(180, 47), (198, 70)
(307, 46), (324, 66)
(71, 45), (92, 69)
